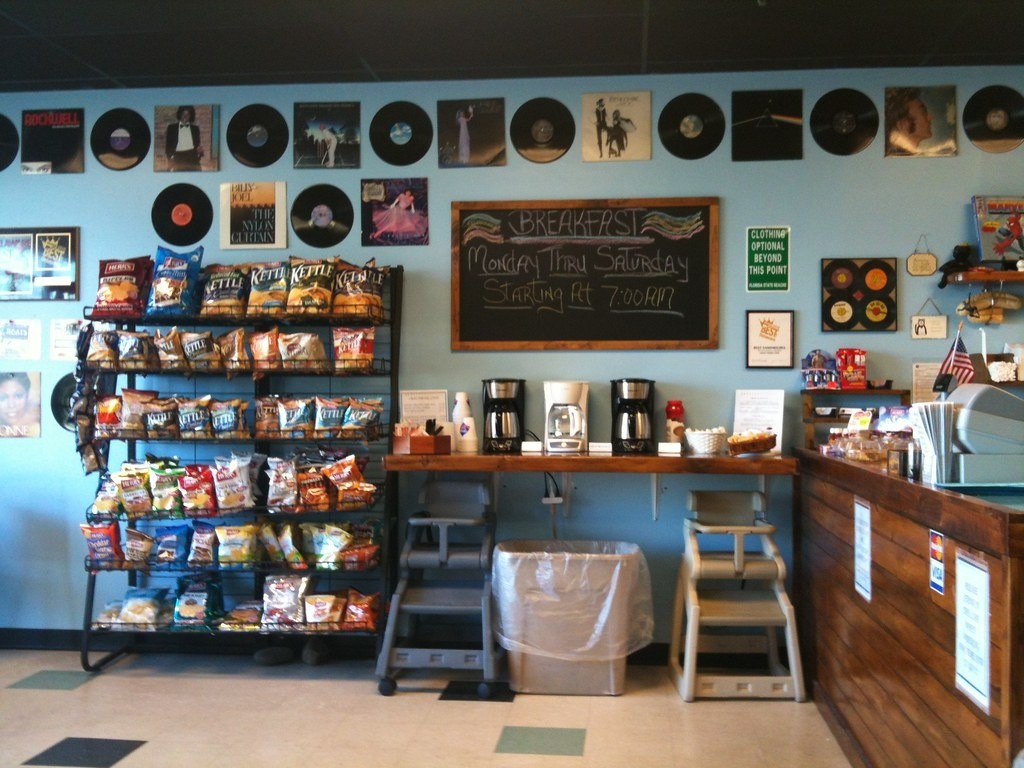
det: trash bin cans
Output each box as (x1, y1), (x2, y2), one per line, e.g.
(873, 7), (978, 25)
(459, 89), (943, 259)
(489, 540), (655, 697)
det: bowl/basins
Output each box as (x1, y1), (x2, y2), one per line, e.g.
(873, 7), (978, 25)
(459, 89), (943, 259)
(683, 431), (729, 454)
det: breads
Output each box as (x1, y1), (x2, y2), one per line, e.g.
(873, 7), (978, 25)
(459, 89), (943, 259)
(956, 291), (1021, 324)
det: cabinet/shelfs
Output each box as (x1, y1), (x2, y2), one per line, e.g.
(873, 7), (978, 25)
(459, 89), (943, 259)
(798, 389), (911, 451)
(79, 263), (403, 671)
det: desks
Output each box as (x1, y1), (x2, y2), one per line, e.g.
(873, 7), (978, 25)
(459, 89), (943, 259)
(382, 452), (802, 515)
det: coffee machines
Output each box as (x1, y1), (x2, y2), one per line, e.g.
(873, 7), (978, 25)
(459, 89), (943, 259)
(543, 381), (591, 452)
(481, 378), (527, 453)
(609, 377), (656, 455)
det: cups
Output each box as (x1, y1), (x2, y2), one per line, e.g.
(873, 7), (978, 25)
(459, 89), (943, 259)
(438, 392), (480, 454)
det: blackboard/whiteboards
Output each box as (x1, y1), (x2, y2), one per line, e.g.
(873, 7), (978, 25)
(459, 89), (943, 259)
(450, 195), (719, 352)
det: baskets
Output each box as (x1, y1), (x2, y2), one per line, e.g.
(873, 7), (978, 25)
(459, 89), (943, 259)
(726, 430), (777, 455)
(682, 432), (728, 455)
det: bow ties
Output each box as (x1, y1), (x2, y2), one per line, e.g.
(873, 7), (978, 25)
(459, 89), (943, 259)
(180, 124), (189, 128)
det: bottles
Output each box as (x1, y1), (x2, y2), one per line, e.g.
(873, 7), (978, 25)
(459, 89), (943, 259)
(829, 427), (856, 446)
(665, 400), (685, 455)
(880, 429), (923, 482)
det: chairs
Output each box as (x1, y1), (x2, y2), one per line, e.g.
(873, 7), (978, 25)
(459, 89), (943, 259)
(376, 480), (505, 699)
(671, 490), (806, 702)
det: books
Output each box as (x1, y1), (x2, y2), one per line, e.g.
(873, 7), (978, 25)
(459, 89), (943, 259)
(972, 195), (1024, 262)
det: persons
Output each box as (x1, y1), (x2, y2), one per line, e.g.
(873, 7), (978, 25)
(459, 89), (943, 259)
(885, 87), (934, 153)
(166, 106), (204, 172)
(0, 372), (41, 426)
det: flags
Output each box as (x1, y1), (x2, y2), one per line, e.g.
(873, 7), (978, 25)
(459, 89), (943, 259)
(939, 336), (976, 384)
